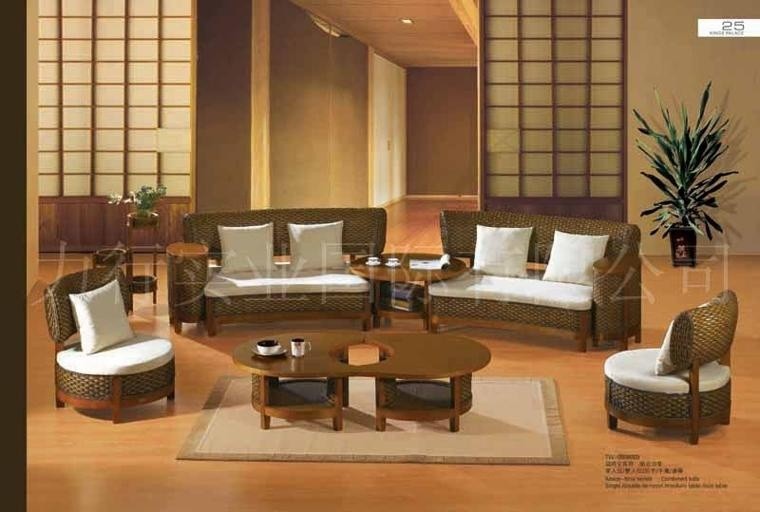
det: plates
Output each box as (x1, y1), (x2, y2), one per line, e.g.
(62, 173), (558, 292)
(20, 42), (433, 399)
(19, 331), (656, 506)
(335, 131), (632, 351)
(365, 261), (381, 266)
(385, 263), (401, 267)
(250, 348), (288, 357)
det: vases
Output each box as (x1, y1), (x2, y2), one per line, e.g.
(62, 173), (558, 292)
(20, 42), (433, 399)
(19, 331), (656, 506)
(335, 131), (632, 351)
(136, 208), (152, 219)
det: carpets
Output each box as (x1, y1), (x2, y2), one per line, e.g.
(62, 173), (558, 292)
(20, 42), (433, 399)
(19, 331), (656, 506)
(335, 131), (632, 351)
(173, 372), (568, 466)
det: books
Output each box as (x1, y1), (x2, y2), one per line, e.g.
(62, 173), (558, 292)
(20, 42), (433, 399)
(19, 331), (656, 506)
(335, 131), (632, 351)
(408, 253), (452, 271)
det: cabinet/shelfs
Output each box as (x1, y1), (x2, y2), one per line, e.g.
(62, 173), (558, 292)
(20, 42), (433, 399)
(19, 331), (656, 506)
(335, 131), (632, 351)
(92, 212), (159, 307)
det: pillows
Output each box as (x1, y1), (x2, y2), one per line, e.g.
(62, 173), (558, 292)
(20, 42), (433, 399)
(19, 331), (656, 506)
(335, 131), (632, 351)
(69, 277), (132, 354)
(470, 224), (534, 279)
(541, 229), (610, 286)
(213, 222), (275, 282)
(286, 220), (348, 276)
(655, 301), (710, 374)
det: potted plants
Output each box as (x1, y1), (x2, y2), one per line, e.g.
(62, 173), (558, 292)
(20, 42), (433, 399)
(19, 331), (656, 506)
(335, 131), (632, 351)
(630, 80), (738, 269)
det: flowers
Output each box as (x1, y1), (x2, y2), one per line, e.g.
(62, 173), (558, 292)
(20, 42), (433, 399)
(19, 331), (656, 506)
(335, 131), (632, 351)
(110, 184), (168, 210)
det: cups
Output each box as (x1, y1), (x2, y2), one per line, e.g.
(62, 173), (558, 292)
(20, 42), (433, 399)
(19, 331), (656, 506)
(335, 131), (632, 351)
(290, 338), (312, 358)
(388, 258), (398, 264)
(368, 256), (379, 263)
(256, 340), (281, 355)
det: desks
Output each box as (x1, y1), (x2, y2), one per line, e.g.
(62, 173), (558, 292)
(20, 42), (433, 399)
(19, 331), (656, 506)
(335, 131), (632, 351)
(349, 250), (465, 330)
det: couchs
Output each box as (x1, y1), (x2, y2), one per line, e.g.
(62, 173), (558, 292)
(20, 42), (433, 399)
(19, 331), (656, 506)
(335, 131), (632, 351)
(164, 205), (386, 337)
(604, 289), (740, 443)
(42, 266), (177, 422)
(428, 207), (641, 351)
